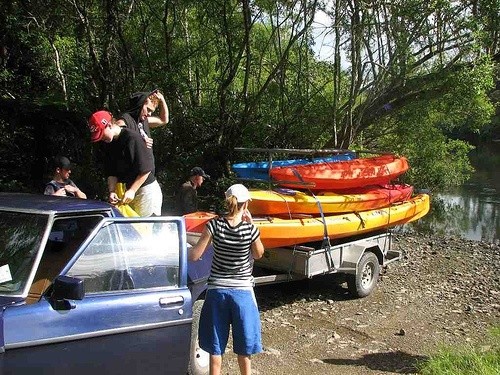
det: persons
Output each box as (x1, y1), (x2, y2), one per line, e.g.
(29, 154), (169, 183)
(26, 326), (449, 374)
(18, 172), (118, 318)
(115, 88), (170, 176)
(176, 166), (210, 216)
(89, 111), (163, 217)
(188, 184), (264, 375)
(44, 157), (87, 199)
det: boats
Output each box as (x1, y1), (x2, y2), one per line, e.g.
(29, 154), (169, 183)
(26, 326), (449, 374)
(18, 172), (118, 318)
(270, 153), (410, 190)
(183, 193), (430, 249)
(247, 183), (414, 216)
(232, 152), (353, 180)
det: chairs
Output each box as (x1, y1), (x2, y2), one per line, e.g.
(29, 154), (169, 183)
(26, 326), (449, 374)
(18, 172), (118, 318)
(14, 224), (106, 295)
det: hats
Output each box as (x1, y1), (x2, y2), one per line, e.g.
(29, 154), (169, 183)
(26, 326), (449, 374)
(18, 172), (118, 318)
(225, 184), (253, 203)
(52, 156), (78, 171)
(190, 167), (210, 178)
(89, 111), (113, 143)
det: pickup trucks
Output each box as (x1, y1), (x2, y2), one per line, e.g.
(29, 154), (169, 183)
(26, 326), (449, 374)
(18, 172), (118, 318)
(0, 190), (212, 375)
